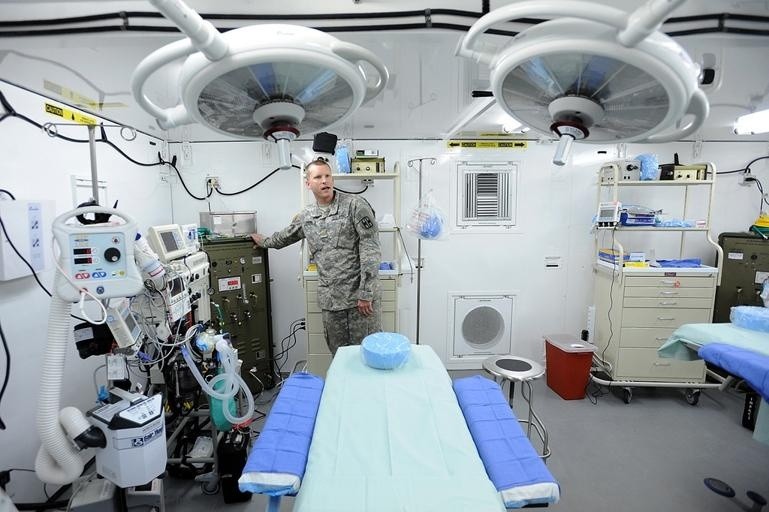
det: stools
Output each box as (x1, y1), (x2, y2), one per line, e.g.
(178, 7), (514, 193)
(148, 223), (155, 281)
(482, 355), (551, 465)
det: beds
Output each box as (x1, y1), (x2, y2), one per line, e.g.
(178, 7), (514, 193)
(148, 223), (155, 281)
(657, 322), (769, 432)
(237, 343), (561, 512)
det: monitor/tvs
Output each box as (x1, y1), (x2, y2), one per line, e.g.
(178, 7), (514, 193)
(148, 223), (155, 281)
(147, 225), (187, 263)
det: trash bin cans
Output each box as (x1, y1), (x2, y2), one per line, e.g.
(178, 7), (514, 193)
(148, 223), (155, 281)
(544, 334), (598, 400)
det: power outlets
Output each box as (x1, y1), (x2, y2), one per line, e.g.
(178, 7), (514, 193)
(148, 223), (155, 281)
(587, 306), (596, 344)
(205, 177), (219, 188)
(739, 175), (757, 186)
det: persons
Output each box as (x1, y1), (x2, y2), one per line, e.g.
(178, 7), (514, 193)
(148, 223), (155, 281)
(249, 159), (384, 361)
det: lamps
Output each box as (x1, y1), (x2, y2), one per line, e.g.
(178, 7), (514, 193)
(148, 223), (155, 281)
(453, 0), (709, 166)
(128, 0), (388, 170)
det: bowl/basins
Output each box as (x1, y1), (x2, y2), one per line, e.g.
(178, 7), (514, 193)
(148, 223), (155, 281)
(735, 305), (769, 331)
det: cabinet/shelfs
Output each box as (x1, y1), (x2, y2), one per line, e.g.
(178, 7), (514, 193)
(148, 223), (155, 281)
(302, 171), (403, 230)
(592, 264), (718, 382)
(304, 277), (399, 379)
(593, 180), (713, 232)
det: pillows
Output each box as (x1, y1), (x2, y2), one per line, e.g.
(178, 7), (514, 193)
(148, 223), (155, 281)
(729, 306), (769, 334)
